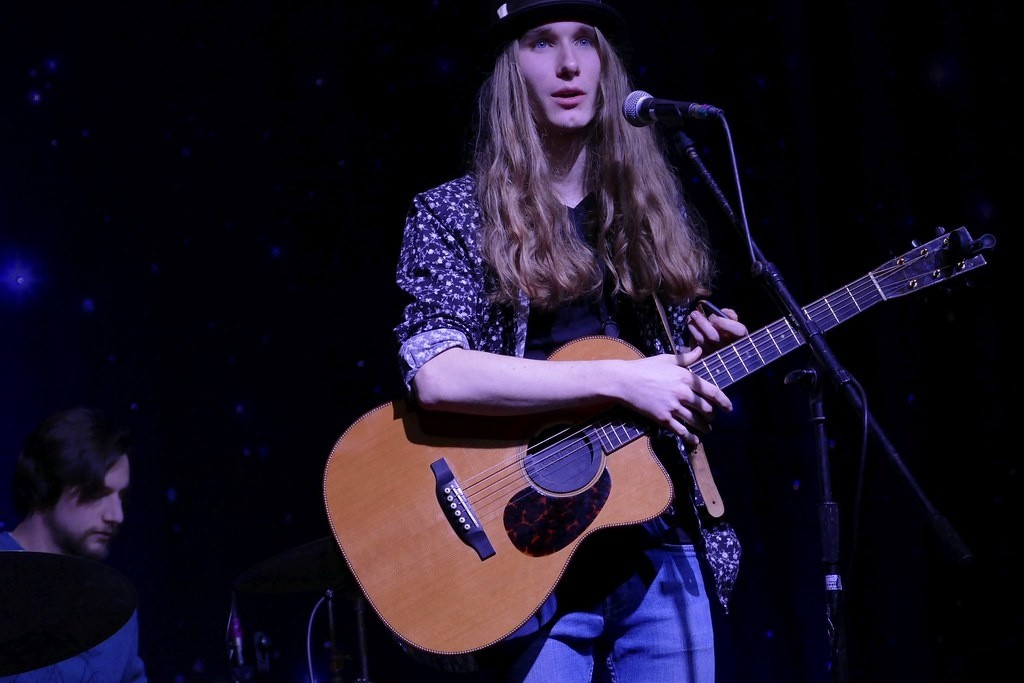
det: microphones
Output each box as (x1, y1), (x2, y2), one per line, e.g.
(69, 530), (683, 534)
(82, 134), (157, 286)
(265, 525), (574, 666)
(621, 90), (723, 127)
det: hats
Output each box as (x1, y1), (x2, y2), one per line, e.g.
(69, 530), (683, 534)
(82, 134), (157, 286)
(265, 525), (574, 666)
(464, 0), (628, 78)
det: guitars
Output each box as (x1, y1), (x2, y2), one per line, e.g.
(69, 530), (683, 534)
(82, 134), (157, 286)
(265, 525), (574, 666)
(324, 218), (1000, 657)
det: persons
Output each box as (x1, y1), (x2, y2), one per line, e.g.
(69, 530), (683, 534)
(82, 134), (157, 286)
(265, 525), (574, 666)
(390, 0), (751, 683)
(0, 404), (152, 683)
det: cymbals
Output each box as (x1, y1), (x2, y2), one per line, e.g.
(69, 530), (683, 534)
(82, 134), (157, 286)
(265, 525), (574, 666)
(0, 550), (139, 679)
